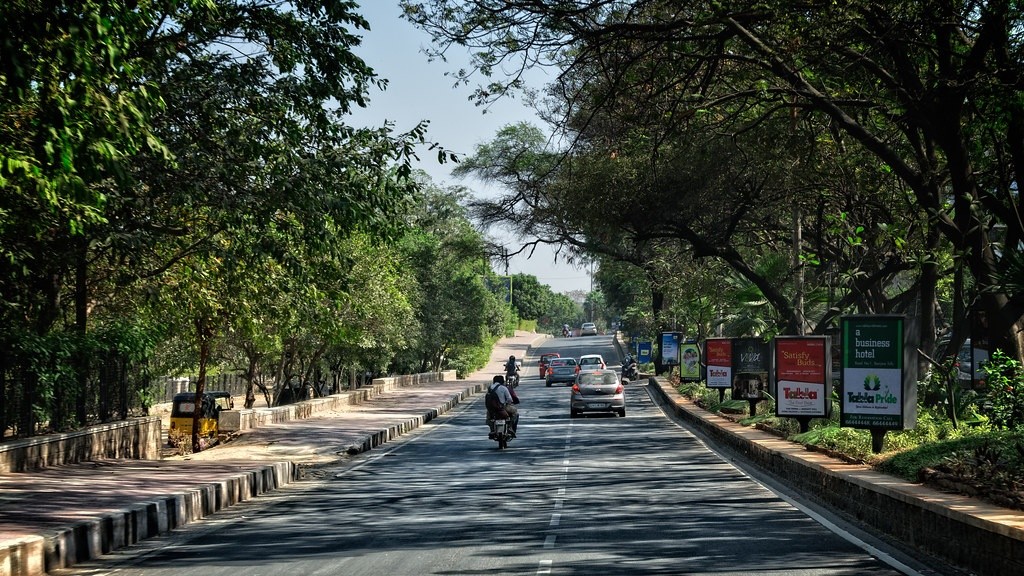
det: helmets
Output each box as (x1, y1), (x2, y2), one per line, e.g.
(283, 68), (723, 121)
(509, 356), (515, 361)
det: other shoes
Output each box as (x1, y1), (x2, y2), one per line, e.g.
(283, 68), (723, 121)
(489, 432), (496, 436)
(489, 435), (495, 439)
(512, 433), (516, 438)
(508, 429), (515, 435)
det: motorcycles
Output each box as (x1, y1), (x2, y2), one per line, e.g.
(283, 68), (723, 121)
(488, 416), (515, 450)
(504, 364), (520, 388)
(564, 330), (567, 337)
(167, 391), (234, 446)
(620, 363), (636, 381)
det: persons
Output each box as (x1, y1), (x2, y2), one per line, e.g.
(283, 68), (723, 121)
(485, 375), (520, 438)
(562, 322), (572, 337)
(504, 355), (521, 387)
(619, 353), (636, 371)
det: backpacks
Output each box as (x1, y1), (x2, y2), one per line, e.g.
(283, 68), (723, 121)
(507, 363), (515, 376)
(629, 356), (636, 364)
(485, 384), (501, 412)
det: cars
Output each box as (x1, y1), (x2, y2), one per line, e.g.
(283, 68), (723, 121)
(538, 352), (608, 387)
(566, 369), (628, 417)
(580, 323), (597, 336)
